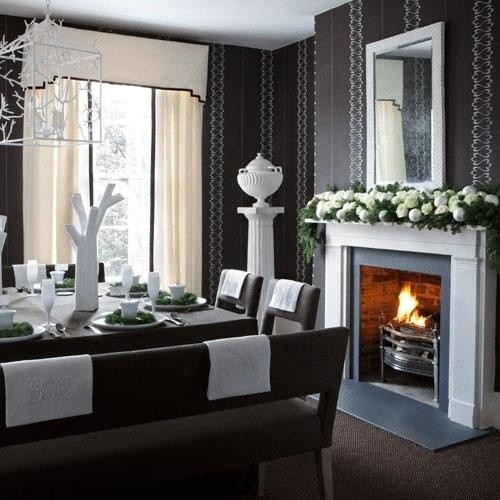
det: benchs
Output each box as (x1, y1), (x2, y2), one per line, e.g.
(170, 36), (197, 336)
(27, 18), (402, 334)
(0, 327), (350, 500)
(2, 263), (105, 288)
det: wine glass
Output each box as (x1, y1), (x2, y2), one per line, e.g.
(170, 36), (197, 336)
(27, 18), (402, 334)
(0, 264), (208, 343)
(26, 259), (39, 298)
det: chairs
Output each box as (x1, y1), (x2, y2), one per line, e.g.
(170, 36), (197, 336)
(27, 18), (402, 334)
(261, 279), (321, 401)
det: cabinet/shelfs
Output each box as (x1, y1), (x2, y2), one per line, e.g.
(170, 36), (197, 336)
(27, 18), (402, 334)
(215, 269), (263, 318)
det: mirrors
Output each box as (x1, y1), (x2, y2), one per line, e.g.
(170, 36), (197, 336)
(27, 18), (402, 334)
(365, 21), (446, 191)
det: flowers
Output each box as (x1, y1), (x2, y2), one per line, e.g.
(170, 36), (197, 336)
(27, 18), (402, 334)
(298, 180), (499, 271)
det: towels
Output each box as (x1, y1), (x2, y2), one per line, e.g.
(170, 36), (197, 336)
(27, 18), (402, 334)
(221, 269), (251, 300)
(269, 279), (309, 313)
(203, 333), (271, 401)
(0, 354), (93, 428)
(12, 264), (47, 289)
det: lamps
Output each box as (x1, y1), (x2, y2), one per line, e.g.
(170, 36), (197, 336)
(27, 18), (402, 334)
(0, 0), (102, 148)
